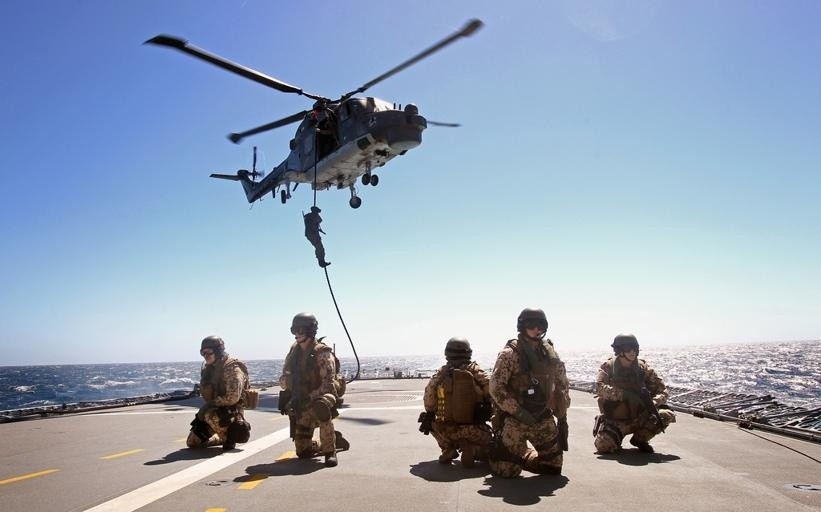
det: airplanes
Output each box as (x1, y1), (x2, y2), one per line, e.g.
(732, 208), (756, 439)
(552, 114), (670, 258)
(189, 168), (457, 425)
(137, 15), (493, 212)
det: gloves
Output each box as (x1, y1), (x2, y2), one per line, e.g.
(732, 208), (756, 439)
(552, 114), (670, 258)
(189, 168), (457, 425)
(622, 389), (652, 428)
(513, 408), (539, 427)
(197, 401), (215, 420)
(285, 396), (310, 416)
(557, 414), (569, 451)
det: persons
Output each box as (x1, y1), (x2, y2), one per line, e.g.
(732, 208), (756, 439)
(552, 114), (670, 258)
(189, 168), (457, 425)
(277, 312), (349, 467)
(592, 332), (676, 455)
(303, 206), (331, 267)
(187, 336), (259, 452)
(418, 336), (494, 467)
(478, 308), (571, 480)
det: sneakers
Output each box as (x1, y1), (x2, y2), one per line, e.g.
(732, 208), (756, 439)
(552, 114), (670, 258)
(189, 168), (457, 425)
(334, 431), (349, 450)
(324, 453), (337, 467)
(222, 439), (235, 449)
(439, 439), (489, 469)
(630, 437), (652, 453)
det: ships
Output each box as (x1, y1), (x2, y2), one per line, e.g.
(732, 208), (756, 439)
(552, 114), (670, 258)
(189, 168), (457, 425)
(0, 369), (820, 512)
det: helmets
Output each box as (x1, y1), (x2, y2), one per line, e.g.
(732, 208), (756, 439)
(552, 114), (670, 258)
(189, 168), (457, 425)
(200, 336), (224, 350)
(291, 312), (317, 330)
(445, 336), (471, 358)
(613, 334), (637, 346)
(518, 308), (548, 332)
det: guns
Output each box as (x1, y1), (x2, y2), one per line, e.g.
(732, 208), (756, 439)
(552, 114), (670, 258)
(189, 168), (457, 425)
(631, 365), (666, 432)
(418, 412), (436, 435)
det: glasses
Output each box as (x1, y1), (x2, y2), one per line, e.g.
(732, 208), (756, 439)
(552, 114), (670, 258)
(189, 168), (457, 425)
(290, 327), (309, 335)
(621, 343), (638, 353)
(199, 349), (214, 356)
(519, 316), (547, 330)
